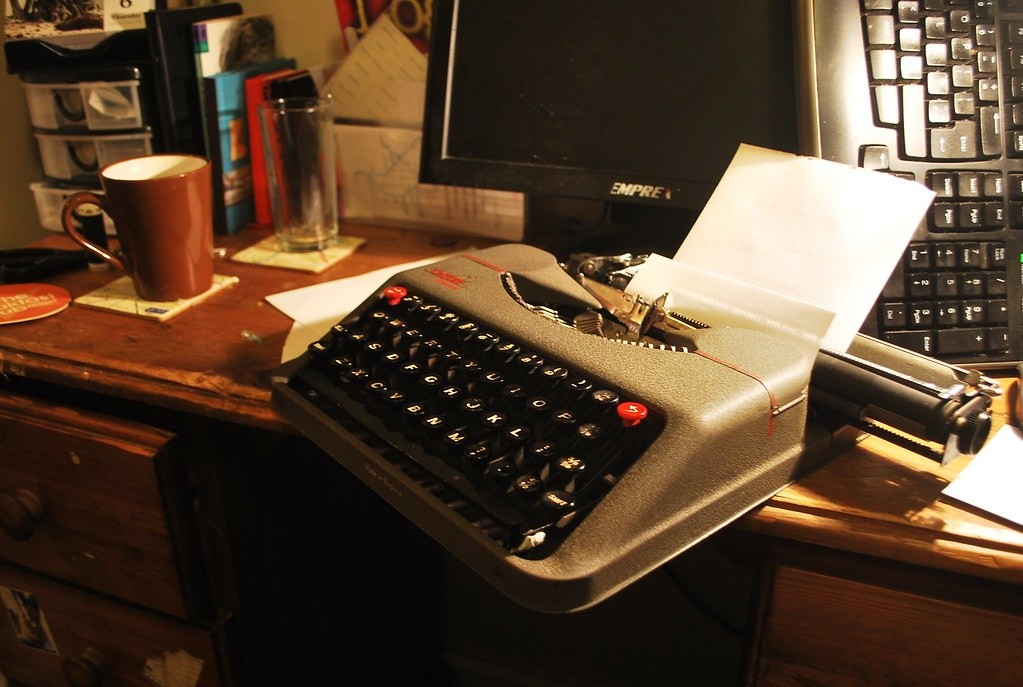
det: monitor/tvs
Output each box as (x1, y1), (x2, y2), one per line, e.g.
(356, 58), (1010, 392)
(415, 0), (799, 262)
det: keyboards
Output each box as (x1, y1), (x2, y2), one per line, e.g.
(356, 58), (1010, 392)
(297, 285), (660, 556)
(791, 0), (1023, 375)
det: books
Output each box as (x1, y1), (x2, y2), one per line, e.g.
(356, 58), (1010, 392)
(144, 3), (318, 238)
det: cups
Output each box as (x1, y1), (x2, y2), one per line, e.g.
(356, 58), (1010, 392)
(257, 96), (339, 254)
(62, 154), (214, 301)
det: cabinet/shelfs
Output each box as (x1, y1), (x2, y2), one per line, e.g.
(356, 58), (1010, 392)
(4, 28), (168, 236)
(0, 214), (1022, 687)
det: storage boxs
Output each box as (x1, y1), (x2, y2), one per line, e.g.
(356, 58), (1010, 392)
(329, 124), (526, 243)
